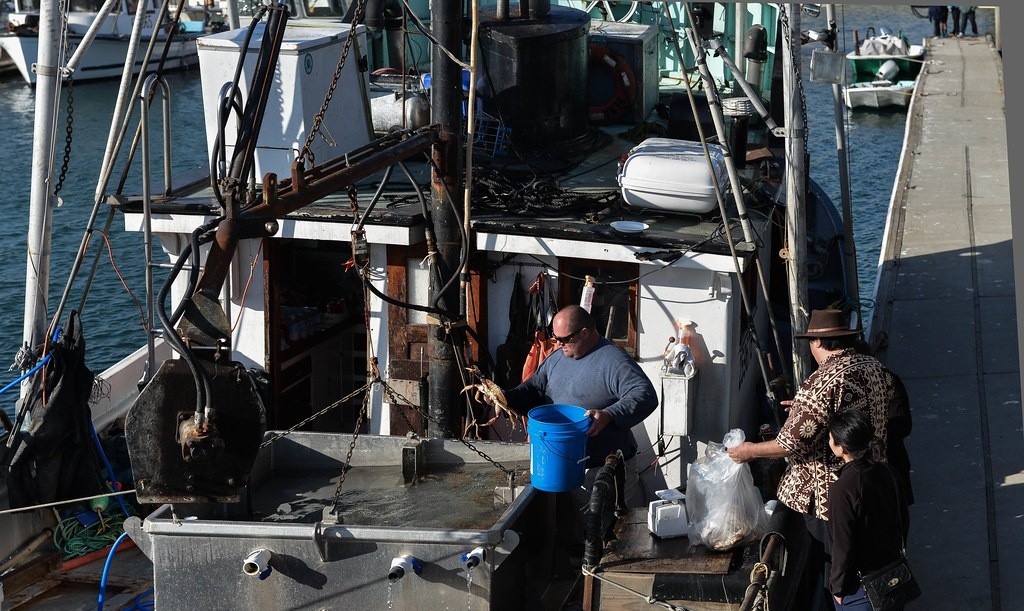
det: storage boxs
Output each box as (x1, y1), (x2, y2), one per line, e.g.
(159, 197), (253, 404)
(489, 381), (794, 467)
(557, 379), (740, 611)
(615, 138), (729, 214)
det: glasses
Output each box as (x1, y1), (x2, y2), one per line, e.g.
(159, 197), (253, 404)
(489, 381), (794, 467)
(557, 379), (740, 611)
(552, 326), (588, 342)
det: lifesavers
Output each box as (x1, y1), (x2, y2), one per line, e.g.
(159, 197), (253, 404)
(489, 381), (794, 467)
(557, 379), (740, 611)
(587, 43), (636, 126)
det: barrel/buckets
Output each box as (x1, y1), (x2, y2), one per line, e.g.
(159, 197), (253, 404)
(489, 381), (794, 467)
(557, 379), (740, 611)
(527, 404), (589, 492)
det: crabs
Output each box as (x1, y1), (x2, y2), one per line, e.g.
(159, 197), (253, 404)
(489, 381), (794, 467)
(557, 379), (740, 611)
(460, 365), (519, 430)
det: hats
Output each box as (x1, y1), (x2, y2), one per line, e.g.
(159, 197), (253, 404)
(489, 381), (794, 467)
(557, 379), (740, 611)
(794, 307), (865, 339)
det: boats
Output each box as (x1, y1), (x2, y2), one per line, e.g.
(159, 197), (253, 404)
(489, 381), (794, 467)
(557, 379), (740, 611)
(0, 0), (216, 91)
(841, 33), (927, 112)
(0, 2), (865, 611)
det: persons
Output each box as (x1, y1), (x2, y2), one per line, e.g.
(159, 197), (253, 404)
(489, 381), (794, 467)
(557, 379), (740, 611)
(823, 411), (911, 611)
(484, 303), (658, 509)
(927, 5), (979, 39)
(726, 308), (913, 550)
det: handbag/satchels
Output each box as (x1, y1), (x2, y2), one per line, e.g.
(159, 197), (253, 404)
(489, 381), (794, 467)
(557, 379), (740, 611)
(860, 556), (923, 611)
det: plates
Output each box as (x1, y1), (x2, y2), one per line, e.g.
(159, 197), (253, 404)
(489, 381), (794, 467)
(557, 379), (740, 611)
(610, 221), (649, 233)
